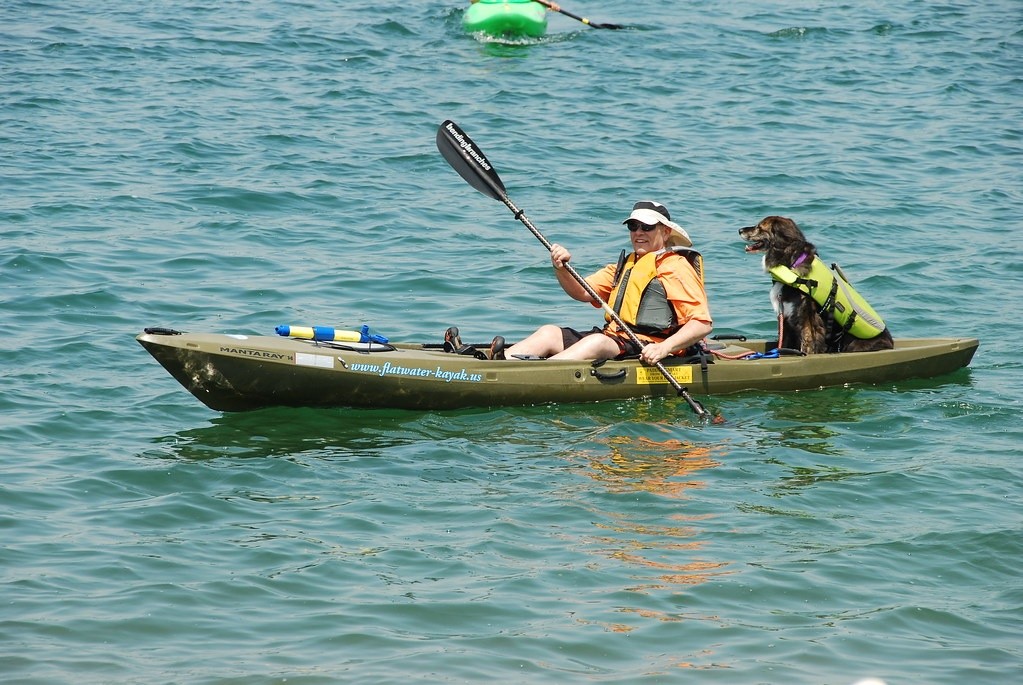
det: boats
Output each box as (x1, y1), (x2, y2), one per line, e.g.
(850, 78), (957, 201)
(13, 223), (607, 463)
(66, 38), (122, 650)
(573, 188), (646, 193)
(135, 324), (983, 412)
(460, 0), (549, 37)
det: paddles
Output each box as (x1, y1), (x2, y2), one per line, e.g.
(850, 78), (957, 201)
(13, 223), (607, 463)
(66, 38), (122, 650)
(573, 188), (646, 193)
(435, 120), (708, 416)
(535, 0), (618, 30)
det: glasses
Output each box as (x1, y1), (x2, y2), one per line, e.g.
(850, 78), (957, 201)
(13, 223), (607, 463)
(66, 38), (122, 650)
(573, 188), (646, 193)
(627, 221), (663, 232)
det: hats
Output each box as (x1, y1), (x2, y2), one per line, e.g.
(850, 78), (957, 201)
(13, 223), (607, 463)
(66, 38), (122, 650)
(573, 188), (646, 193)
(621, 201), (692, 247)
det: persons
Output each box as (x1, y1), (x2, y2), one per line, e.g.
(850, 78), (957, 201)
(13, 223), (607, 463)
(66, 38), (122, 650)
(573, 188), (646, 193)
(445, 201), (712, 366)
(539, 0), (561, 13)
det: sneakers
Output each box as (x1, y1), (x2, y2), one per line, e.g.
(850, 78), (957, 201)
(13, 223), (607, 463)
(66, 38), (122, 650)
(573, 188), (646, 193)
(488, 335), (506, 360)
(444, 326), (464, 354)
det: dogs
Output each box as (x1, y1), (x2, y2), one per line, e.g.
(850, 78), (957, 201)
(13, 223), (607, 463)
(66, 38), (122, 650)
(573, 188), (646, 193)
(738, 216), (893, 351)
